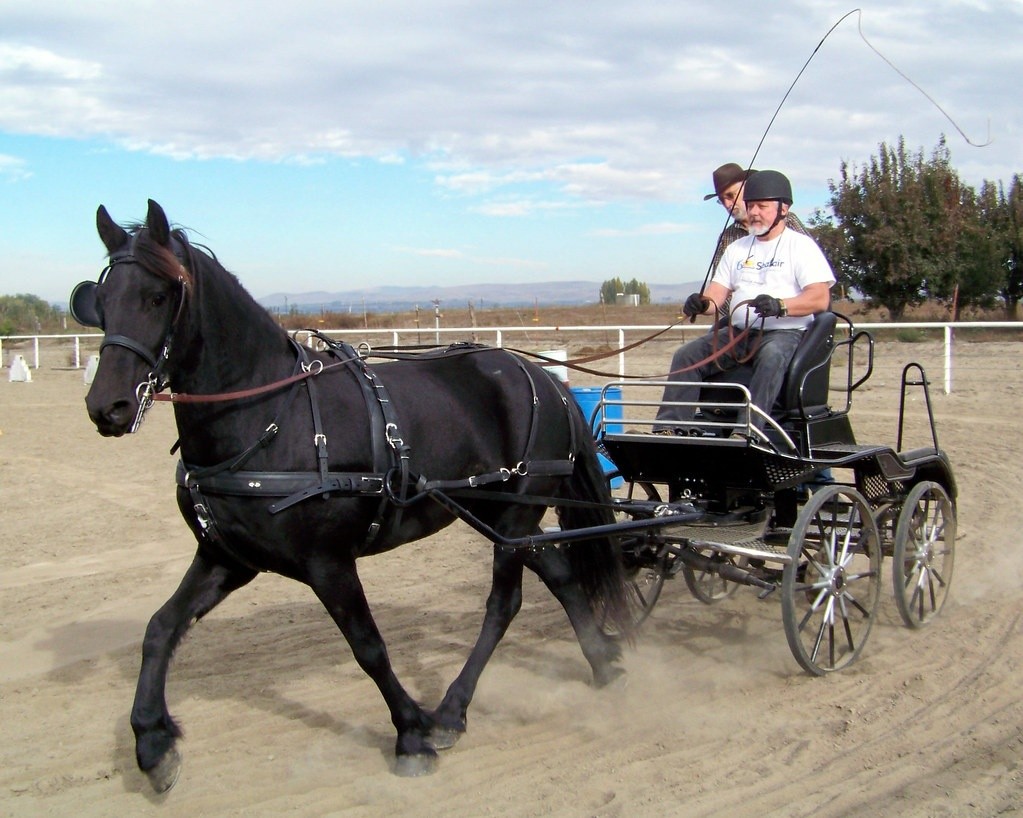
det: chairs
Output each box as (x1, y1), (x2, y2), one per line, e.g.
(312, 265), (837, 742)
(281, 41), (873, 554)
(696, 311), (837, 420)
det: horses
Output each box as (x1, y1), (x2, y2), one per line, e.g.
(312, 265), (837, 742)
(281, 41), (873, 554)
(84, 198), (640, 797)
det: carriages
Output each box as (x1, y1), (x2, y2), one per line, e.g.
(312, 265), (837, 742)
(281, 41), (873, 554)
(67, 195), (965, 798)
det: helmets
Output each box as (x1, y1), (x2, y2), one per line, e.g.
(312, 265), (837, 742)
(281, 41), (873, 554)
(741, 169), (793, 205)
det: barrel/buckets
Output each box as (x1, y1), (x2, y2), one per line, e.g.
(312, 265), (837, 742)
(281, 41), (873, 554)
(573, 387), (624, 490)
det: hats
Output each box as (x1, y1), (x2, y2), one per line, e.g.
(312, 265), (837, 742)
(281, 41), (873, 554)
(704, 161), (758, 201)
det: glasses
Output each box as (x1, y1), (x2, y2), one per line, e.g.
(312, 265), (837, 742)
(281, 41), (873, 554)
(717, 183), (744, 204)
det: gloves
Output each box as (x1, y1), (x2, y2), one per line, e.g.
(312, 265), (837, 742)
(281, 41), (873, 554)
(682, 292), (710, 317)
(747, 294), (787, 318)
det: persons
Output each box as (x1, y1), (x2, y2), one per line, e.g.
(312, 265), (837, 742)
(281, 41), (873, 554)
(704, 163), (814, 318)
(625, 170), (836, 443)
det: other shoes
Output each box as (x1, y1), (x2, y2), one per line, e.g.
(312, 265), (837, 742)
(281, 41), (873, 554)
(625, 428), (678, 436)
(728, 433), (747, 440)
(812, 486), (843, 502)
